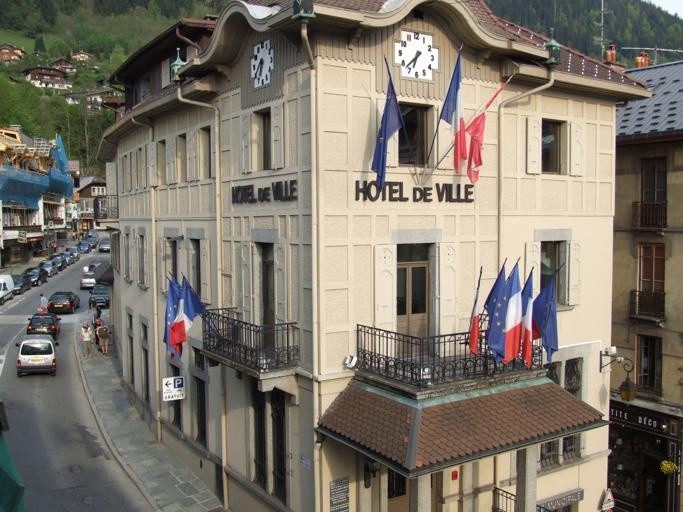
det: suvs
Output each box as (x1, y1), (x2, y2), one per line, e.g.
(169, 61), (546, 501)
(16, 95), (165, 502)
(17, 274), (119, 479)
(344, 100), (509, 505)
(88, 283), (110, 309)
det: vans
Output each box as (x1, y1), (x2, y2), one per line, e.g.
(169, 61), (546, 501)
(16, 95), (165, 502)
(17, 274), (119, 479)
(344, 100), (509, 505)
(15, 333), (60, 377)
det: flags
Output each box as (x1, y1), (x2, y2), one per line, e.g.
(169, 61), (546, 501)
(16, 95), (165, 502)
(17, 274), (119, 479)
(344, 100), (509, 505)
(439, 53), (468, 176)
(371, 74), (404, 192)
(467, 79), (510, 188)
(163, 275), (204, 361)
(471, 264), (558, 370)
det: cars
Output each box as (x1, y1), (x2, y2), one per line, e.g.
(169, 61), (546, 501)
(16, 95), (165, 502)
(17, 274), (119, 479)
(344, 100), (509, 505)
(24, 311), (62, 341)
(0, 228), (99, 309)
(99, 238), (111, 253)
(46, 290), (81, 316)
(86, 260), (103, 271)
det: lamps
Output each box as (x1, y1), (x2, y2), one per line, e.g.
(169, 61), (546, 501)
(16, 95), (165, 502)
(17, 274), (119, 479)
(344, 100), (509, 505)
(600, 345), (638, 402)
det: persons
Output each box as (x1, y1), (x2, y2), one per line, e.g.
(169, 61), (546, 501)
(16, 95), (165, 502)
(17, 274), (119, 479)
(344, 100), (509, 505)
(39, 293), (48, 313)
(80, 306), (109, 359)
(88, 291), (92, 309)
